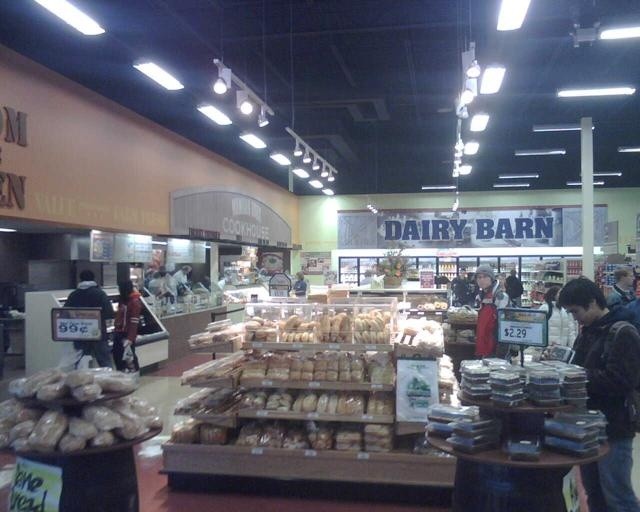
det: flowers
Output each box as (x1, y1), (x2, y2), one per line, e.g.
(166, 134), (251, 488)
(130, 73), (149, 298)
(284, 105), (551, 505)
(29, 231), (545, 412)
(380, 246), (414, 278)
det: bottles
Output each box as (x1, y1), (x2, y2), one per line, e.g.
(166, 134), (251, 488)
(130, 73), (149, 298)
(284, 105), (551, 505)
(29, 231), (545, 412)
(596, 263), (635, 286)
(520, 256), (582, 307)
(340, 257), (520, 290)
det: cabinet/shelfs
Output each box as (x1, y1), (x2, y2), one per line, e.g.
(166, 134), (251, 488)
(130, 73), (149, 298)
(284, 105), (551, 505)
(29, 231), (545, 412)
(416, 256), (584, 306)
(339, 257), (380, 287)
(159, 321), (460, 503)
(1, 385), (163, 512)
(425, 387), (611, 512)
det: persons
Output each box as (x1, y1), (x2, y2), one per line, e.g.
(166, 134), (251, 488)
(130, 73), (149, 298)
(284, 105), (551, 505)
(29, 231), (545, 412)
(474, 265), (519, 359)
(606, 268), (636, 308)
(557, 275), (640, 512)
(63, 270), (116, 369)
(144, 264), (193, 300)
(293, 271), (307, 298)
(452, 267), (524, 306)
(538, 286), (579, 348)
(113, 278), (142, 373)
(624, 274), (640, 331)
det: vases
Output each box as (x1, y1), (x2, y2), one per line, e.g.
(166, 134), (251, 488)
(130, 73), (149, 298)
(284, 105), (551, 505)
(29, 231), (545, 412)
(383, 274), (403, 289)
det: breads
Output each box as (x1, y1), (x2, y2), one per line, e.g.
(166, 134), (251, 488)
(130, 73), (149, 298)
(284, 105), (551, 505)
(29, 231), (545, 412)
(172, 311), (391, 452)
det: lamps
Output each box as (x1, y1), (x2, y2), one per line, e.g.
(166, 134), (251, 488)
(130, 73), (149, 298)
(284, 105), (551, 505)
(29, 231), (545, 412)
(212, 58), (275, 130)
(452, 0), (480, 176)
(285, 127), (338, 183)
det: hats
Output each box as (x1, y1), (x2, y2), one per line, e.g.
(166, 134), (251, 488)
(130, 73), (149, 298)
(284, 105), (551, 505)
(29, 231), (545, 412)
(475, 262), (497, 281)
